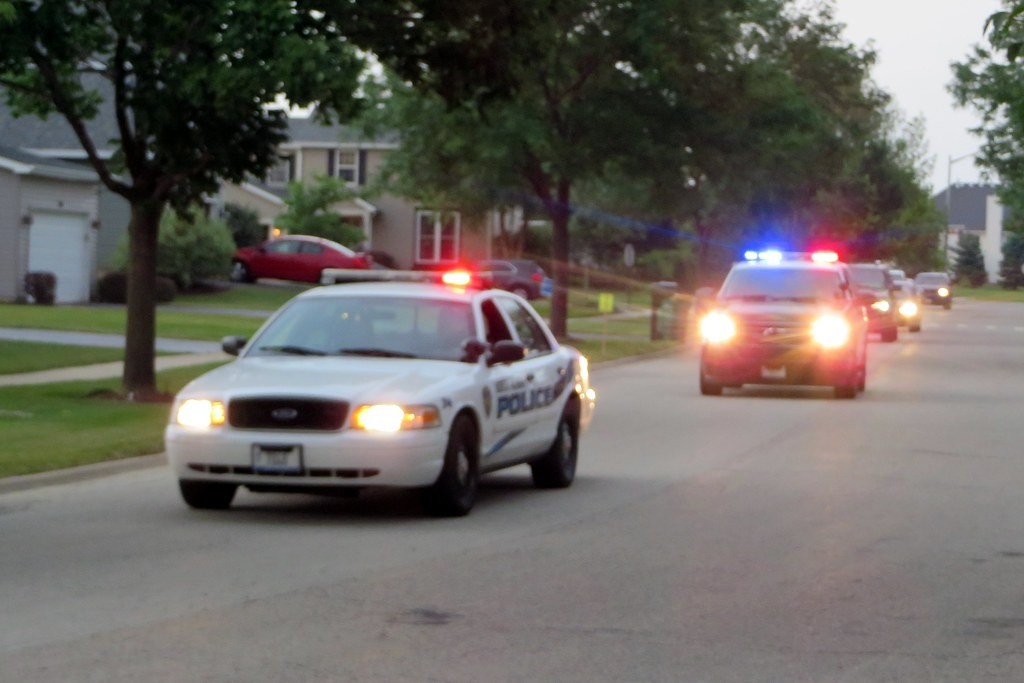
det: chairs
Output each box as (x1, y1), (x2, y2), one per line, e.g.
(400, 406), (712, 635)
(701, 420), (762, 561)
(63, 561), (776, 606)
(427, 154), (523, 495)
(431, 306), (476, 361)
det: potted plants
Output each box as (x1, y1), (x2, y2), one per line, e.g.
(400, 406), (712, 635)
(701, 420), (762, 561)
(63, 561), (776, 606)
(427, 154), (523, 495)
(24, 272), (55, 303)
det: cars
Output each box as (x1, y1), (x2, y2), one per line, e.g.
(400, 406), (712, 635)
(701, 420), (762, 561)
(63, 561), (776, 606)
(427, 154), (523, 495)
(229, 236), (375, 284)
(470, 256), (546, 301)
(162, 270), (594, 520)
(889, 271), (921, 331)
(699, 250), (875, 398)
(914, 272), (953, 309)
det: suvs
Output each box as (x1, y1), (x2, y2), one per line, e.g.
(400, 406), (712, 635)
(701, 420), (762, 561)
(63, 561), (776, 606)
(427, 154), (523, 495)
(844, 261), (898, 342)
